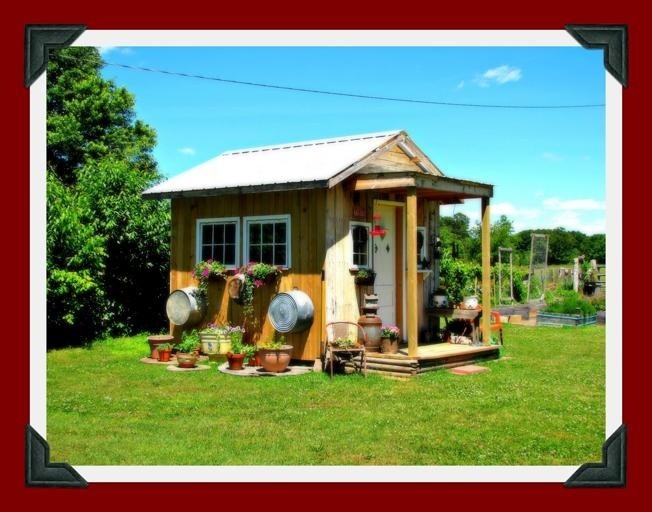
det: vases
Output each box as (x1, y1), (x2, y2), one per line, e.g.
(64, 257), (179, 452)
(249, 351), (262, 366)
(201, 334), (243, 355)
(148, 335), (173, 359)
(380, 336), (397, 354)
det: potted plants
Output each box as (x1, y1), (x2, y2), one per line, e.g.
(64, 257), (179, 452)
(174, 330), (201, 368)
(434, 325), (447, 343)
(258, 329), (294, 371)
(432, 288), (448, 307)
(158, 344), (172, 362)
(226, 343), (254, 370)
(463, 288), (478, 309)
(354, 269), (375, 286)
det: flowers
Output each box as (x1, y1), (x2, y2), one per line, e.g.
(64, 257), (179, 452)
(201, 323), (246, 333)
(238, 261), (282, 318)
(191, 258), (225, 310)
(381, 323), (401, 337)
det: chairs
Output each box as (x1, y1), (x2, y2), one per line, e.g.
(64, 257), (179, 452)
(326, 321), (368, 378)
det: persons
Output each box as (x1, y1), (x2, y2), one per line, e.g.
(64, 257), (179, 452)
(587, 268), (600, 296)
